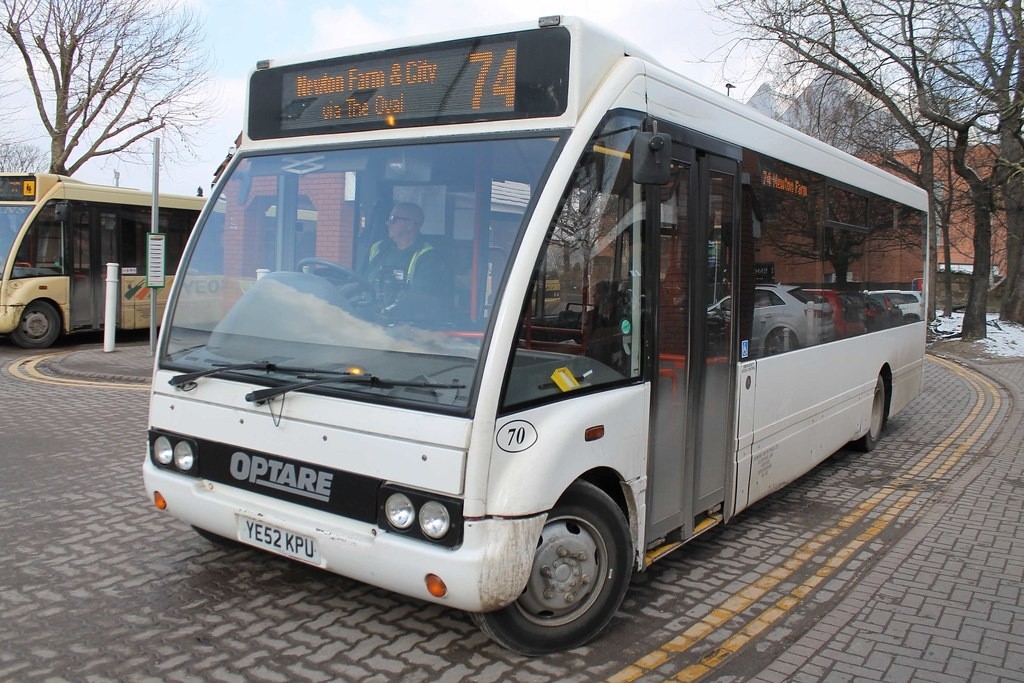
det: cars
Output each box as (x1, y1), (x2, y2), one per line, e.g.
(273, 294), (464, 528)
(705, 279), (923, 362)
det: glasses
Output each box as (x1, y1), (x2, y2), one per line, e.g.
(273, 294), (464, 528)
(389, 216), (412, 224)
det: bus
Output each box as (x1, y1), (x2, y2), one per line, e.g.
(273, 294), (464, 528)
(142, 12), (930, 658)
(0, 172), (223, 349)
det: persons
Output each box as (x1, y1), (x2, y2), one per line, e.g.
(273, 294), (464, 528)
(357, 201), (442, 322)
(578, 280), (632, 361)
